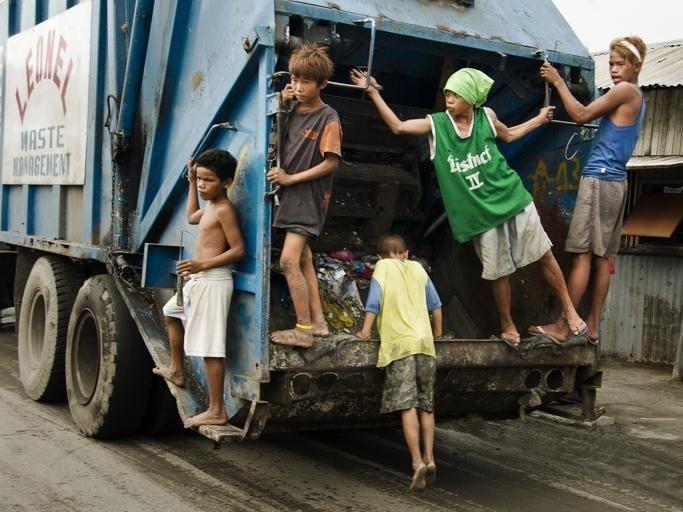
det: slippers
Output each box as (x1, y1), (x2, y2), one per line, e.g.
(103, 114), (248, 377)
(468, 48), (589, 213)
(499, 317), (601, 351)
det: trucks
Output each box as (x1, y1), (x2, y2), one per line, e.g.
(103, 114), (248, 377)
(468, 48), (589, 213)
(1, 1), (604, 443)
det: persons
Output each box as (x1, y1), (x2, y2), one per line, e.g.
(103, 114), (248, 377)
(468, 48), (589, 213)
(527, 33), (648, 345)
(346, 64), (589, 347)
(152, 146), (245, 432)
(269, 37), (345, 348)
(358, 234), (445, 492)
